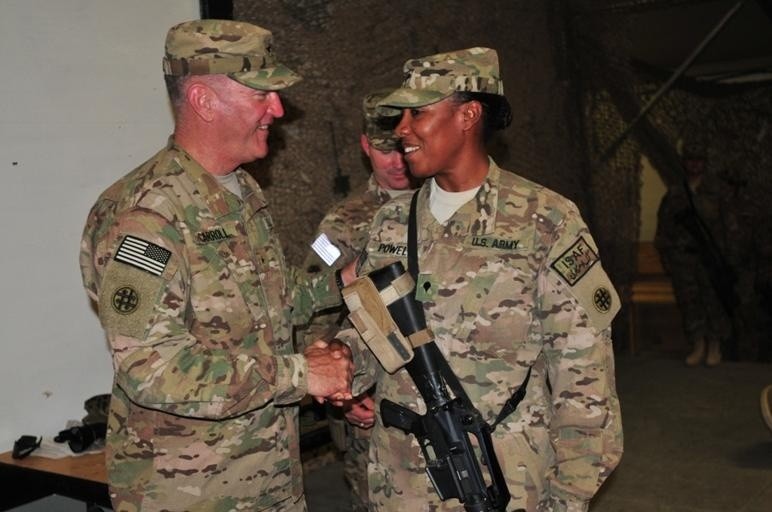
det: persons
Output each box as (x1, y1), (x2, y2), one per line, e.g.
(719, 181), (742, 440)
(655, 143), (739, 367)
(81, 22), (360, 510)
(316, 47), (624, 512)
(307, 89), (424, 511)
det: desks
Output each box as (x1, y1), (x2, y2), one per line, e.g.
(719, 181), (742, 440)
(0, 448), (112, 511)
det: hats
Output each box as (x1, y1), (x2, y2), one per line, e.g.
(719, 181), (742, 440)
(360, 88), (401, 151)
(162, 18), (304, 92)
(375, 47), (504, 117)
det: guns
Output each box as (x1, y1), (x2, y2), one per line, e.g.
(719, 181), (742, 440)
(368, 262), (511, 512)
